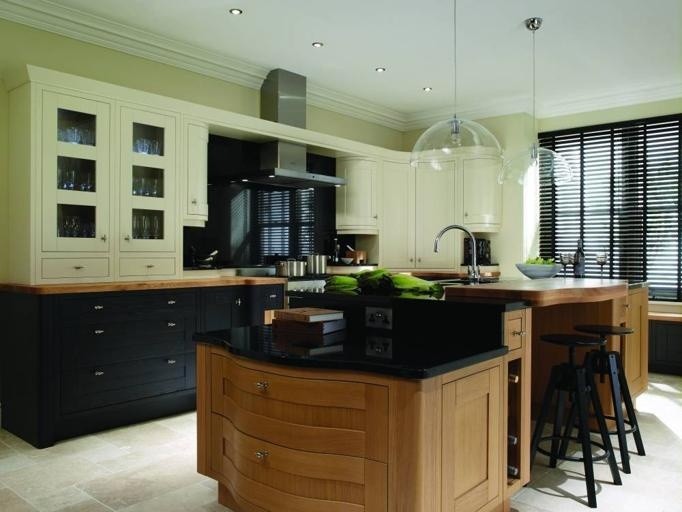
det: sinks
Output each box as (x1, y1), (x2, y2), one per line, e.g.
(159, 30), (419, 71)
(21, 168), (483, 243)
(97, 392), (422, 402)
(439, 280), (470, 285)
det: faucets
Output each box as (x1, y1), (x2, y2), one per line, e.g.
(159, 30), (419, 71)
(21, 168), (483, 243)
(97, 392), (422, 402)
(432, 221), (479, 280)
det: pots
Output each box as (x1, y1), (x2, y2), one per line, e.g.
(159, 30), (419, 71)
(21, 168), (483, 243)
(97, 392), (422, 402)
(276, 261), (306, 277)
(300, 254), (329, 274)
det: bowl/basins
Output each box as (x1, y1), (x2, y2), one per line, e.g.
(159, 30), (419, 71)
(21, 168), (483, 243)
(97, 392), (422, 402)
(516, 263), (563, 279)
(341, 258), (353, 265)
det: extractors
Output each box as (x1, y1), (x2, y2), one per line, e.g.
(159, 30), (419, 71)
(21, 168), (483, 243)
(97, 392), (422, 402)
(208, 69), (347, 189)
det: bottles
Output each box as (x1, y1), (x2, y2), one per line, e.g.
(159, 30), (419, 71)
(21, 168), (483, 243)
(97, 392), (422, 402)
(332, 239), (339, 265)
(574, 240), (585, 277)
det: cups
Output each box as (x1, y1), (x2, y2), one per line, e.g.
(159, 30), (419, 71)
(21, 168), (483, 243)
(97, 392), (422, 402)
(134, 177), (158, 196)
(134, 137), (161, 155)
(57, 128), (94, 146)
(58, 168), (92, 191)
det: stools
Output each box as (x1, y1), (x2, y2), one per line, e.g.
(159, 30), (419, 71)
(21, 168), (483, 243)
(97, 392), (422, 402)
(558, 325), (645, 474)
(522, 333), (623, 508)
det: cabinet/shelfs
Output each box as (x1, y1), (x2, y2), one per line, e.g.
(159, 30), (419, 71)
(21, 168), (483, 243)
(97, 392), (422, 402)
(628, 288), (648, 414)
(198, 283), (284, 336)
(464, 158), (503, 233)
(379, 160), (464, 273)
(196, 344), (508, 512)
(37, 284), (197, 449)
(4, 64), (209, 284)
(335, 156), (378, 235)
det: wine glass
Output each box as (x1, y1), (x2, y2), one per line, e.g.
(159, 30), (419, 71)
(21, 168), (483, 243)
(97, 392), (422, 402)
(57, 216), (95, 238)
(596, 253), (607, 279)
(133, 214), (161, 239)
(568, 254), (574, 265)
(560, 253), (569, 277)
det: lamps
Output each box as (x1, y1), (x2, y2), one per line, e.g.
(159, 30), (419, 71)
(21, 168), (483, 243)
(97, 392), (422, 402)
(496, 18), (573, 186)
(409, 0), (503, 172)
